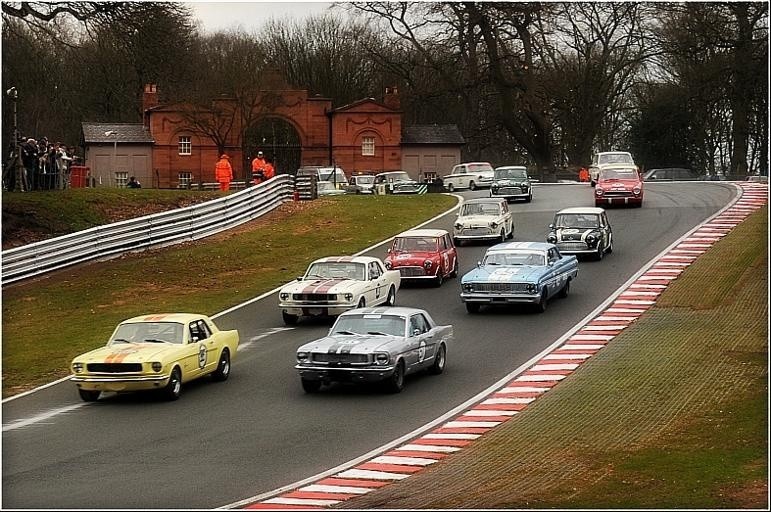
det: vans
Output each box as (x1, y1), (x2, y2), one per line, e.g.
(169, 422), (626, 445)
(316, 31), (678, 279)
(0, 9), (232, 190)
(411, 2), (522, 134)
(297, 165), (348, 190)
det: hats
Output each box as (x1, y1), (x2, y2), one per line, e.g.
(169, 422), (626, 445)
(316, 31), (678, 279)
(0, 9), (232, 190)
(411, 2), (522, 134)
(221, 154), (229, 159)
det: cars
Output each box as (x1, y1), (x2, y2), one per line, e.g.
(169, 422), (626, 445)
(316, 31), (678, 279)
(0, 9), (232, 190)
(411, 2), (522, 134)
(70, 313), (239, 401)
(385, 229), (458, 287)
(277, 254), (401, 324)
(443, 162), (495, 192)
(295, 306), (453, 393)
(452, 198), (513, 245)
(345, 171), (416, 194)
(460, 241), (579, 311)
(589, 150), (644, 207)
(644, 168), (701, 182)
(547, 207), (613, 261)
(489, 166), (532, 202)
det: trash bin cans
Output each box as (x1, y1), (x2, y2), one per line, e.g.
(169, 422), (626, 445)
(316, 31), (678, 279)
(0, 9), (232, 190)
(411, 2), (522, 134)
(70, 166), (87, 188)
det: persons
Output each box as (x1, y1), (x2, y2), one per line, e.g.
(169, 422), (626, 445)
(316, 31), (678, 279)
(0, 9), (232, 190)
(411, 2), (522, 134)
(251, 152), (266, 185)
(2, 131), (86, 191)
(424, 175), (435, 183)
(564, 152), (569, 162)
(562, 159), (568, 169)
(265, 159), (275, 180)
(579, 167), (588, 182)
(433, 174), (445, 192)
(215, 154), (234, 192)
(126, 176), (141, 188)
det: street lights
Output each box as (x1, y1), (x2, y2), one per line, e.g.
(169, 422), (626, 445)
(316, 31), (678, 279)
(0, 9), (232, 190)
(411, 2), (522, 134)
(104, 130), (117, 186)
(7, 87), (24, 193)
